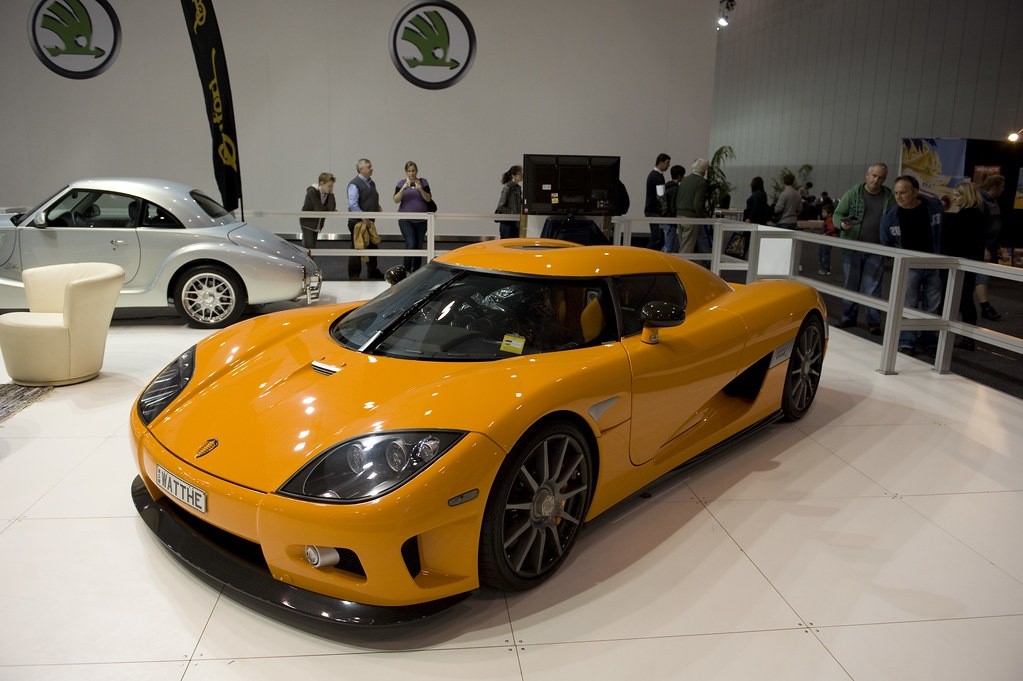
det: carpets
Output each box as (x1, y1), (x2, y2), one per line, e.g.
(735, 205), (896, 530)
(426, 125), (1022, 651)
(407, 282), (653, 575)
(0, 383), (57, 424)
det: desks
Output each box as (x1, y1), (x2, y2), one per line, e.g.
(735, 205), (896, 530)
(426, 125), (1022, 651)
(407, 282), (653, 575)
(797, 219), (824, 232)
(714, 209), (744, 222)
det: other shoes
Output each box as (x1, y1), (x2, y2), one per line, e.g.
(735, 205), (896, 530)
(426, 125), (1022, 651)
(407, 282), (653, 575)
(367, 272), (384, 280)
(898, 346), (911, 355)
(817, 269), (829, 275)
(349, 275), (360, 280)
(954, 338), (975, 352)
(924, 340), (937, 356)
(980, 305), (1002, 320)
(834, 319), (857, 328)
(799, 265), (803, 271)
(870, 324), (883, 335)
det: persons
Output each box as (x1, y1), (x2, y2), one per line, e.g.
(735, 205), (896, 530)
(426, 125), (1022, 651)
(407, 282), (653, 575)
(830, 163), (896, 336)
(775, 173), (803, 272)
(299, 173), (339, 250)
(742, 175), (768, 260)
(497, 164), (524, 239)
(642, 154), (671, 251)
(674, 157), (709, 253)
(660, 165), (685, 253)
(878, 174), (944, 353)
(799, 181), (837, 275)
(346, 159), (386, 281)
(392, 160), (433, 278)
(944, 174), (1012, 353)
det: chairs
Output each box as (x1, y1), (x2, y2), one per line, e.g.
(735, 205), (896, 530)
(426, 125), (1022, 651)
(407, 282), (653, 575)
(126, 199), (170, 226)
(543, 281), (632, 331)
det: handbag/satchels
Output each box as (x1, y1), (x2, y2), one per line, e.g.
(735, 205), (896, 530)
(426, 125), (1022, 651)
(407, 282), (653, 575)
(724, 232), (748, 260)
(494, 185), (511, 223)
(419, 177), (437, 212)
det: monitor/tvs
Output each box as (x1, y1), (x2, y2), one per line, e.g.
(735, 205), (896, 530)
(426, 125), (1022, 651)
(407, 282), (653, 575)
(523, 154), (620, 216)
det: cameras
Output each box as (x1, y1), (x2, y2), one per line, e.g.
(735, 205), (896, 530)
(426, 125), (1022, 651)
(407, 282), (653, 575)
(410, 182), (415, 187)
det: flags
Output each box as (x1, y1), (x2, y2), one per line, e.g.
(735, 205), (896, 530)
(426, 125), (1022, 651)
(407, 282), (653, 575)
(180, 0), (242, 213)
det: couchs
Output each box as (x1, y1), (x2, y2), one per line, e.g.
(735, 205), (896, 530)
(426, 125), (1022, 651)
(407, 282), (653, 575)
(0, 261), (126, 386)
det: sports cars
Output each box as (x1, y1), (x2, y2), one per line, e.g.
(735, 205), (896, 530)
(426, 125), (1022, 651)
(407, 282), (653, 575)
(128, 236), (832, 632)
(0, 175), (325, 329)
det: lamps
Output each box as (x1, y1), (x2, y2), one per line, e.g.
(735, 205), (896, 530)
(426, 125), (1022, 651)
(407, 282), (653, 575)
(715, 0), (737, 29)
